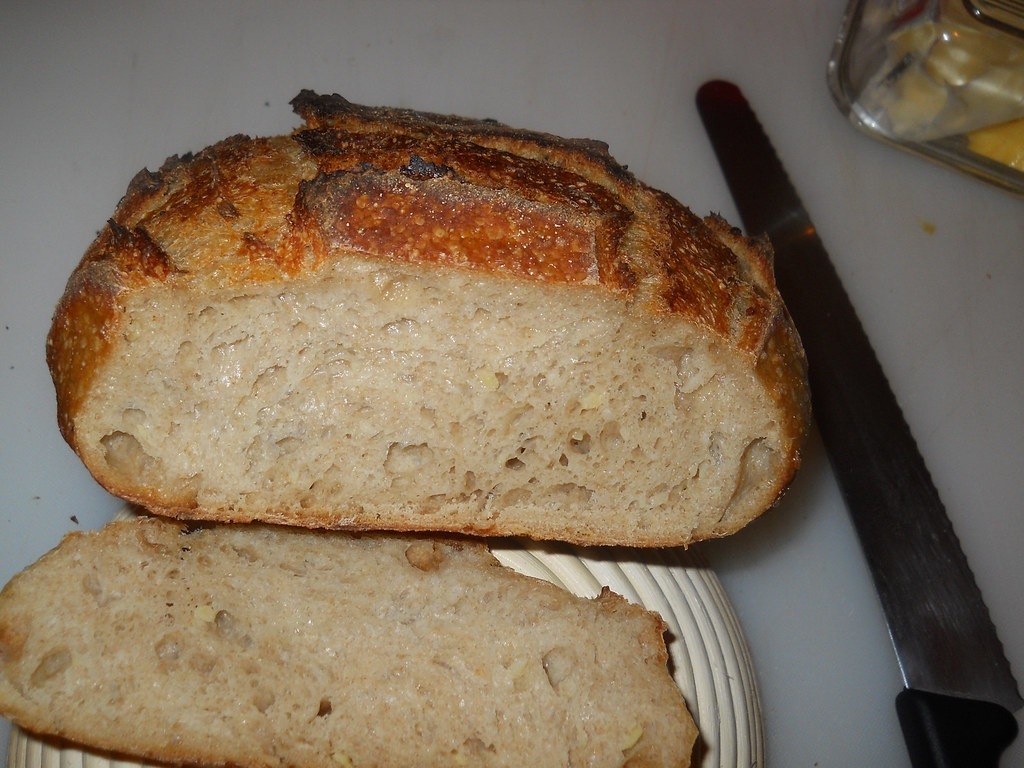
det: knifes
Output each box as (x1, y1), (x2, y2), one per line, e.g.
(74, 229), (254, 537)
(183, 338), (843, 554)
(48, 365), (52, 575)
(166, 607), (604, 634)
(695, 80), (1023, 768)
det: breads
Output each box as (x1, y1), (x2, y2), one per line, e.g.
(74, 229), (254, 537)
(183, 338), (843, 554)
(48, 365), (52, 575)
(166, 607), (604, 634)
(48, 88), (812, 545)
(1, 519), (698, 768)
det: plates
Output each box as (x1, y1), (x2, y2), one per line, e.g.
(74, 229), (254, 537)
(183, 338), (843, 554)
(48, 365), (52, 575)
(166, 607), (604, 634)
(827, 0), (1024, 193)
(12, 502), (763, 768)
